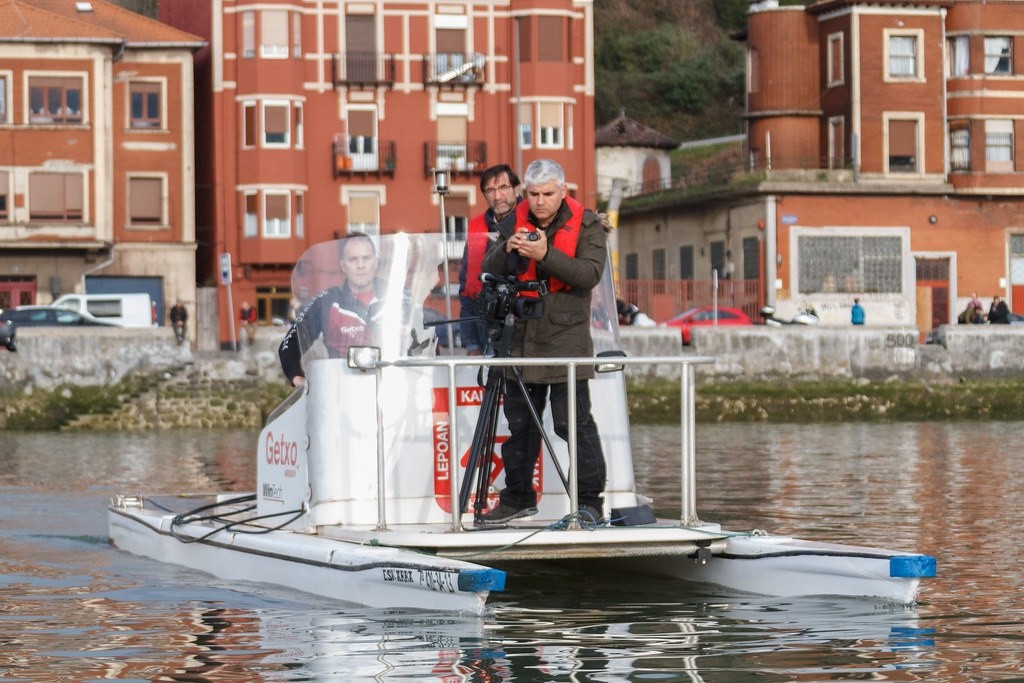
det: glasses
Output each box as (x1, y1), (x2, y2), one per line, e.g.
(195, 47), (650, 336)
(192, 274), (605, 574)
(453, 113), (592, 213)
(484, 184), (513, 197)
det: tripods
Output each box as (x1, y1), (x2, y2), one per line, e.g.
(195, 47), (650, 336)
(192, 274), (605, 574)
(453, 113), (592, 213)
(424, 308), (578, 529)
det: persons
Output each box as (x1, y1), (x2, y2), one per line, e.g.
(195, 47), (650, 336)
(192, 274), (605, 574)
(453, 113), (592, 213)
(479, 159), (607, 529)
(981, 315), (990, 325)
(851, 298), (865, 325)
(988, 297), (1009, 325)
(460, 164), (525, 354)
(279, 232), (439, 387)
(239, 302), (257, 326)
(958, 306), (982, 325)
(169, 302), (188, 345)
(967, 292), (982, 310)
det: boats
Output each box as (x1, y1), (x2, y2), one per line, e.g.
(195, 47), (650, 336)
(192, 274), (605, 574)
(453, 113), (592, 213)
(104, 167), (939, 609)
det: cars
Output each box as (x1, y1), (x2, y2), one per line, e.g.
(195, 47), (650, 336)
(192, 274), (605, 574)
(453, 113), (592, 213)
(661, 303), (755, 348)
(0, 304), (122, 348)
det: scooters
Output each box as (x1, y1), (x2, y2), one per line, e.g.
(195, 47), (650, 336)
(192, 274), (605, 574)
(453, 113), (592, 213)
(759, 299), (823, 329)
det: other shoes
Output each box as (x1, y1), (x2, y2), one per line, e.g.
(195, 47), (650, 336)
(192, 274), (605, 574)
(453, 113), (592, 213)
(474, 503), (538, 523)
(563, 503), (604, 525)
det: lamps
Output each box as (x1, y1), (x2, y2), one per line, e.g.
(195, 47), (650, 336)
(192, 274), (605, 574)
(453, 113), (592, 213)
(346, 345), (379, 373)
(593, 349), (628, 374)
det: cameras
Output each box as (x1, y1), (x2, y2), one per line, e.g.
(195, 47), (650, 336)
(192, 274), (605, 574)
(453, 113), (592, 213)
(522, 232), (540, 241)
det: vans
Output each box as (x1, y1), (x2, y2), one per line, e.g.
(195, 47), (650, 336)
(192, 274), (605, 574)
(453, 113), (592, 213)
(30, 293), (152, 329)
